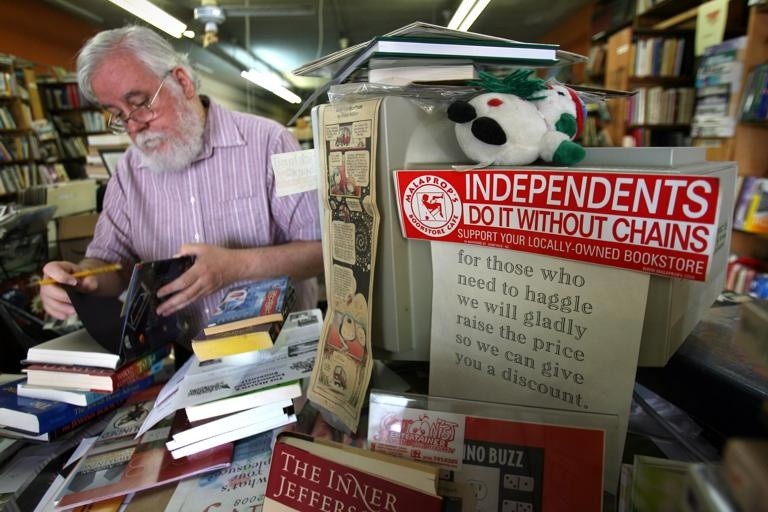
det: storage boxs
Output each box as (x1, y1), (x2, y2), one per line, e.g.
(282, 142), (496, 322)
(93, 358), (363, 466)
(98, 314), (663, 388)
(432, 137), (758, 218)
(52, 212), (102, 264)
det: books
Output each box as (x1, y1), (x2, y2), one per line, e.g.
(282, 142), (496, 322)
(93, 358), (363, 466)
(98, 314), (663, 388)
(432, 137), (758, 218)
(584, 37), (696, 148)
(1, 54), (132, 239)
(287, 20), (562, 126)
(1, 257), (441, 510)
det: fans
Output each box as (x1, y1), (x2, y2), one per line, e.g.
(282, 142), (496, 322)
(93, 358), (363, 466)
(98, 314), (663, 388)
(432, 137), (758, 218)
(170, 0), (319, 50)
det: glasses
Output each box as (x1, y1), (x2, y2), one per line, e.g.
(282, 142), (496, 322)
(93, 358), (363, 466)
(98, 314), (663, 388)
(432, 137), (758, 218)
(105, 69), (174, 136)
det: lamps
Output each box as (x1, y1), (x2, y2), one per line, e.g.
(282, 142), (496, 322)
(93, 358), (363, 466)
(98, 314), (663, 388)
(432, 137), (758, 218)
(237, 50), (303, 108)
(440, 0), (494, 33)
(107, 0), (197, 42)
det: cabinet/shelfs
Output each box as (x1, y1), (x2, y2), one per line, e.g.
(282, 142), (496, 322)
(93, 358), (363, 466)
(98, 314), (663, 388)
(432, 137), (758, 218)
(0, 53), (69, 207)
(521, 1), (767, 272)
(29, 66), (137, 219)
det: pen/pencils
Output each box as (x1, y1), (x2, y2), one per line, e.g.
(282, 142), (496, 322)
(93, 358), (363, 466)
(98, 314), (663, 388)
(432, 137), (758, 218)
(26, 264), (123, 288)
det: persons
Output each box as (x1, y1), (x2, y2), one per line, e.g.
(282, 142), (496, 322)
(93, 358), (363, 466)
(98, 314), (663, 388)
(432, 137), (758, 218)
(40, 25), (324, 368)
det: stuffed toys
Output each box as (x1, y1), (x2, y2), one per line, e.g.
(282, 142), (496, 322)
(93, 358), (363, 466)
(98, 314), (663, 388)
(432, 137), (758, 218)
(449, 81), (586, 166)
